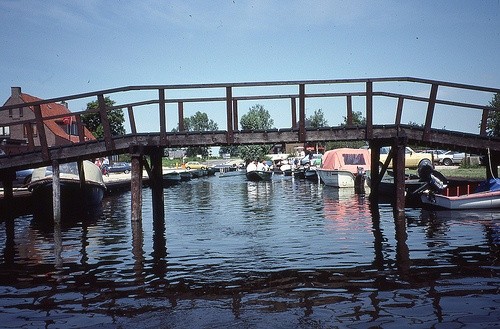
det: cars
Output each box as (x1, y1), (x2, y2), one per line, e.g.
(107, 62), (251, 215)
(368, 147), (432, 169)
(420, 149), (484, 166)
(0, 167), (52, 187)
(300, 154), (323, 166)
(103, 162), (131, 174)
(185, 161), (208, 170)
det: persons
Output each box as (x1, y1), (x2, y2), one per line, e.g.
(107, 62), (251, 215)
(95, 158), (102, 169)
(423, 165), (448, 196)
(101, 157), (109, 177)
(262, 164), (269, 173)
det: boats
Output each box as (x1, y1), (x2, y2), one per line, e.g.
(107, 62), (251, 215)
(271, 154), (324, 179)
(315, 148), (372, 188)
(247, 169), (274, 181)
(365, 170), (428, 197)
(419, 185), (500, 210)
(28, 159), (107, 221)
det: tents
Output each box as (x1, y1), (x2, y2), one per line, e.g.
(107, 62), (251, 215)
(322, 148), (371, 173)
